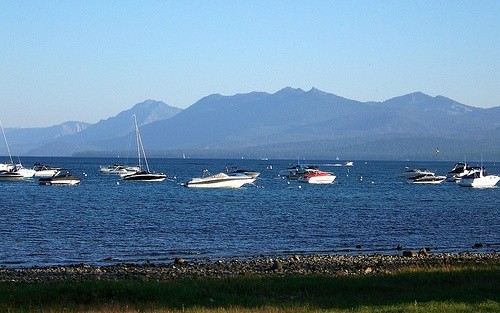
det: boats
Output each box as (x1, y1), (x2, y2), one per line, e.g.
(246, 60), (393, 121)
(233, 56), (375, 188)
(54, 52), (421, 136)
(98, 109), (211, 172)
(181, 166), (261, 189)
(39, 168), (80, 186)
(446, 152), (500, 187)
(279, 156), (337, 184)
(0, 122), (61, 181)
(100, 112), (167, 182)
(406, 171), (448, 185)
